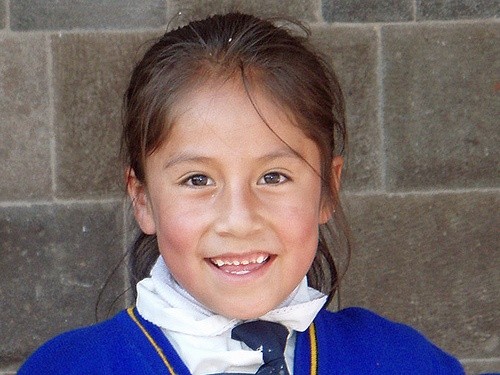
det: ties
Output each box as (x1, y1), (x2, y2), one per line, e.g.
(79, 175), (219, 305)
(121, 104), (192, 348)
(232, 320), (289, 375)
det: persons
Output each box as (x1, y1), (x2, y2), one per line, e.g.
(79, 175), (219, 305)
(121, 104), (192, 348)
(13, 14), (468, 374)
